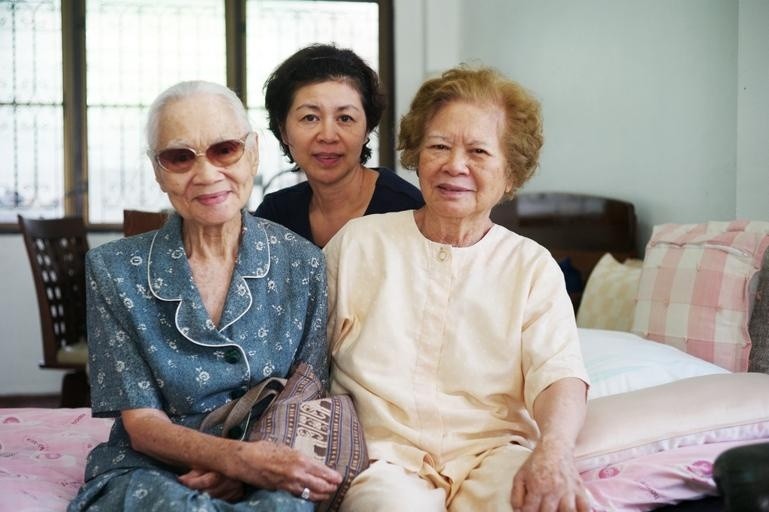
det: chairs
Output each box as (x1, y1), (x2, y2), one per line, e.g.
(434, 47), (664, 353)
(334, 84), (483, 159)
(16, 213), (92, 408)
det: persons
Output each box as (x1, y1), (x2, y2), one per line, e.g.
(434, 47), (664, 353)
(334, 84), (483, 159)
(321, 59), (593, 512)
(66, 78), (344, 512)
(252, 42), (427, 249)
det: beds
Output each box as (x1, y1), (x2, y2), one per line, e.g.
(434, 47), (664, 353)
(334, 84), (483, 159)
(0, 250), (768, 511)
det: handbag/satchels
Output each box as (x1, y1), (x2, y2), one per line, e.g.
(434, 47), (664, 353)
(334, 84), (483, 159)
(243, 363), (369, 512)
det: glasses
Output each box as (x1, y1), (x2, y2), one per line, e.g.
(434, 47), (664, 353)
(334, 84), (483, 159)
(151, 132), (250, 175)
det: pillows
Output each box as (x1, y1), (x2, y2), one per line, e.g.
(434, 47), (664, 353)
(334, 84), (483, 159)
(573, 372), (766, 474)
(573, 222), (765, 374)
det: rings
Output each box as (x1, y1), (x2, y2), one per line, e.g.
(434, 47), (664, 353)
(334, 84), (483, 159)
(300, 489), (313, 501)
